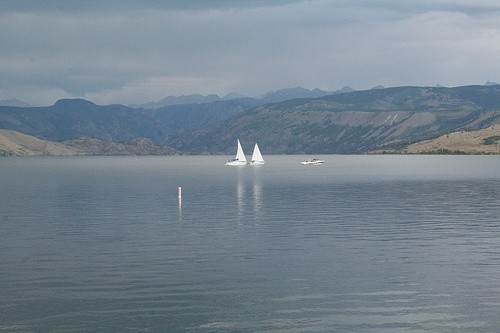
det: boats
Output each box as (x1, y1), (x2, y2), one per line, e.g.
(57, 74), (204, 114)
(301, 159), (324, 165)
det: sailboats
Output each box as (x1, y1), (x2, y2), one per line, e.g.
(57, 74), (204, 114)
(248, 142), (265, 166)
(226, 139), (247, 165)
